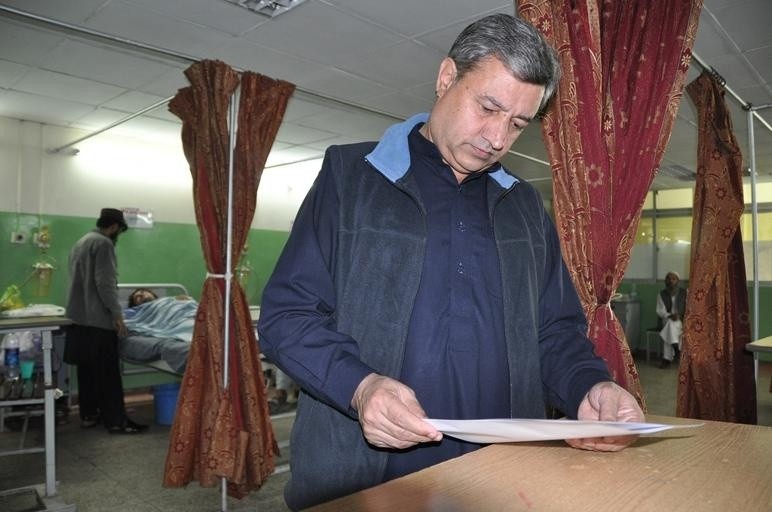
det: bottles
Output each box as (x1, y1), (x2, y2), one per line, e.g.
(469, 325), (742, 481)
(3, 332), (19, 381)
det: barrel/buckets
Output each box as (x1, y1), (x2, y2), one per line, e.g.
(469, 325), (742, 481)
(150, 381), (181, 425)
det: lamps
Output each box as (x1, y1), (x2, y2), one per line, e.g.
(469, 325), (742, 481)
(1, 262), (53, 314)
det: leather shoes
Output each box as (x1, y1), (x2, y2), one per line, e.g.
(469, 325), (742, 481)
(83, 410), (104, 427)
(108, 415), (148, 435)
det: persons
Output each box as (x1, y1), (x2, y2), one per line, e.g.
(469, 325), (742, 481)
(127, 287), (158, 307)
(61, 206), (150, 435)
(255, 10), (650, 510)
(655, 269), (689, 369)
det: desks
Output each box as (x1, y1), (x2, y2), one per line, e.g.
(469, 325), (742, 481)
(297, 411), (771, 510)
(0, 305), (74, 497)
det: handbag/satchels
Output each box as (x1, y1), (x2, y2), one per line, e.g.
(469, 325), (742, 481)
(63, 323), (95, 365)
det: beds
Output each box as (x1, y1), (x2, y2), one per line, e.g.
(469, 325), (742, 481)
(115, 283), (297, 454)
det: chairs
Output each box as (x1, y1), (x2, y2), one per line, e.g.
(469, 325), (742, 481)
(646, 315), (664, 364)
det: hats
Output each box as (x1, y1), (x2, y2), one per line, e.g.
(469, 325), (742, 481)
(100, 209), (128, 231)
